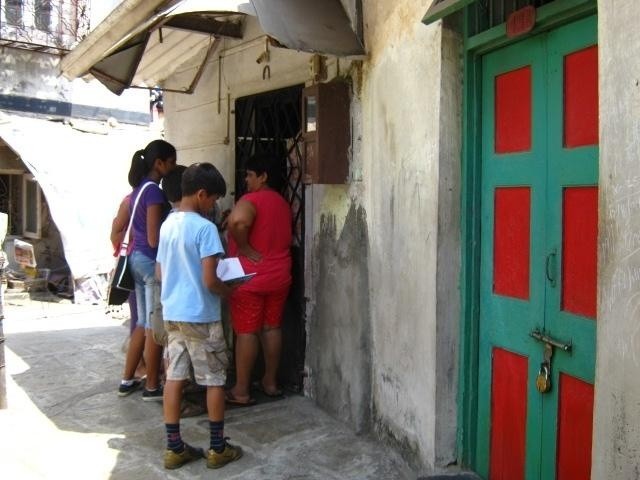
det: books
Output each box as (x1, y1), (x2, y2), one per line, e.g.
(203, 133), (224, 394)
(216, 256), (257, 285)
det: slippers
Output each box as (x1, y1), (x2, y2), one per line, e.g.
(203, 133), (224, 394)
(223, 388), (257, 406)
(250, 380), (285, 397)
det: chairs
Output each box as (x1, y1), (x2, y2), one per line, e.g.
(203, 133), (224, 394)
(13, 239), (52, 292)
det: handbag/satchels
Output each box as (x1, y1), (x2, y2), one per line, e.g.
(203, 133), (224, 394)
(106, 255), (134, 305)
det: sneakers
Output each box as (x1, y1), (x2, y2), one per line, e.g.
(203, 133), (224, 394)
(164, 442), (203, 469)
(206, 437), (242, 469)
(117, 376), (146, 397)
(141, 383), (164, 401)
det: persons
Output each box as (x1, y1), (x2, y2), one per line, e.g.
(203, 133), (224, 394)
(224, 151), (290, 405)
(153, 161), (244, 468)
(113, 142), (237, 418)
